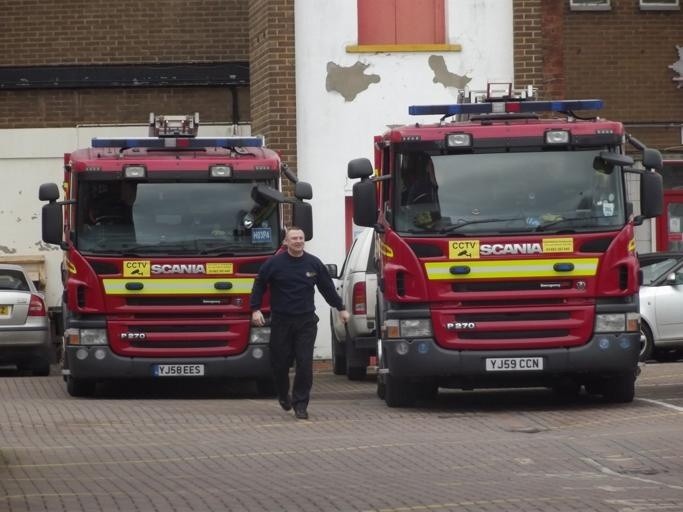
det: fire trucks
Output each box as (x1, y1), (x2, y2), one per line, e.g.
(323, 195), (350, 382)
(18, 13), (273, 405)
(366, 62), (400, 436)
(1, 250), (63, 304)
(38, 113), (312, 396)
(347, 81), (663, 408)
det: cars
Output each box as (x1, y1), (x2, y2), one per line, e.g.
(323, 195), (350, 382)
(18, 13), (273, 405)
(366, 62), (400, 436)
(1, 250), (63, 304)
(637, 256), (682, 361)
(0, 263), (51, 378)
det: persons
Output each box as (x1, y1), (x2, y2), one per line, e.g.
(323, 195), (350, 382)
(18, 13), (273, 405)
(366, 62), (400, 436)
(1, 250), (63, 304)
(250, 225), (352, 418)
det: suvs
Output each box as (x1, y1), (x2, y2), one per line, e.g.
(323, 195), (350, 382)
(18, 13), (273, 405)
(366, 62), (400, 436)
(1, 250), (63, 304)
(327, 225), (377, 381)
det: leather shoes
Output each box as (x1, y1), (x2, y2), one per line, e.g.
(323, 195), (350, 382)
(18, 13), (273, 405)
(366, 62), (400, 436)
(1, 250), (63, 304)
(294, 407), (309, 419)
(278, 393), (292, 410)
